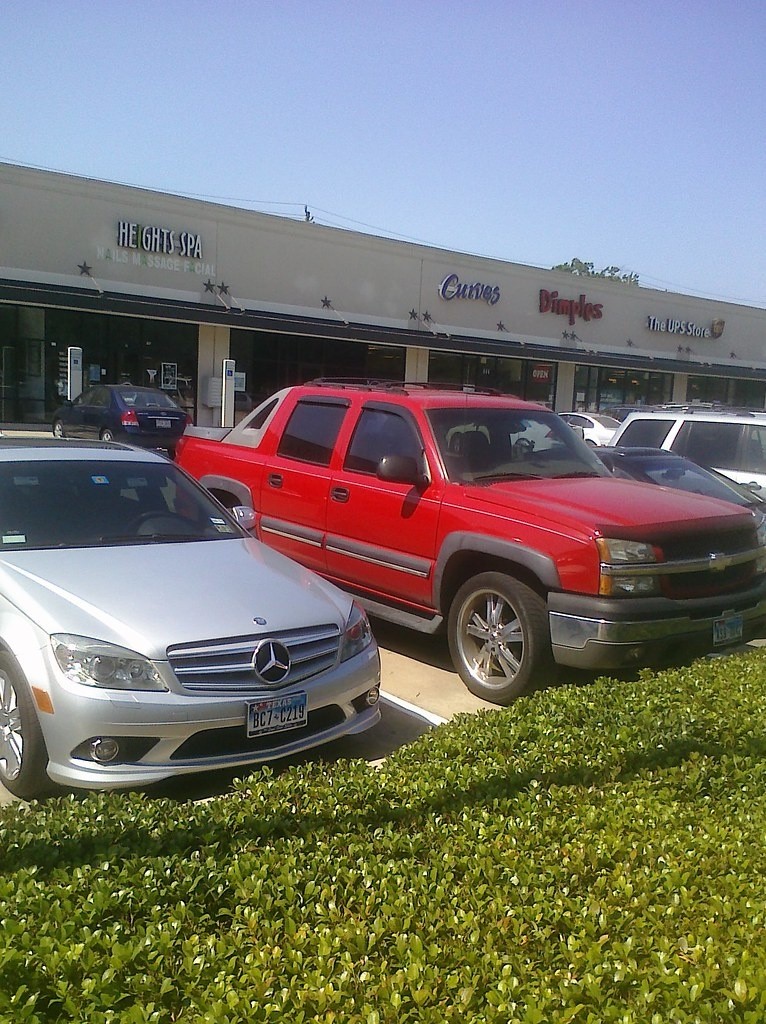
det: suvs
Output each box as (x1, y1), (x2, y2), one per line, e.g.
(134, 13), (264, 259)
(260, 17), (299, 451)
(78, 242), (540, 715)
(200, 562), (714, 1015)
(597, 404), (766, 502)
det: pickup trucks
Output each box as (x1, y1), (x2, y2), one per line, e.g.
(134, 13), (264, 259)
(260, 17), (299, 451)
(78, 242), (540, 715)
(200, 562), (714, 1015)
(175, 373), (766, 706)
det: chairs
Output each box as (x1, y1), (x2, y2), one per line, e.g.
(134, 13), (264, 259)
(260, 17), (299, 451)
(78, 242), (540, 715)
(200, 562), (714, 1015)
(449, 431), (503, 474)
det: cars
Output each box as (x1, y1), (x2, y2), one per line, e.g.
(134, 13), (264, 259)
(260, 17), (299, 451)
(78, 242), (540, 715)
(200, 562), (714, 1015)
(582, 440), (766, 545)
(440, 414), (585, 461)
(0, 431), (386, 804)
(554, 410), (621, 450)
(52, 385), (189, 459)
